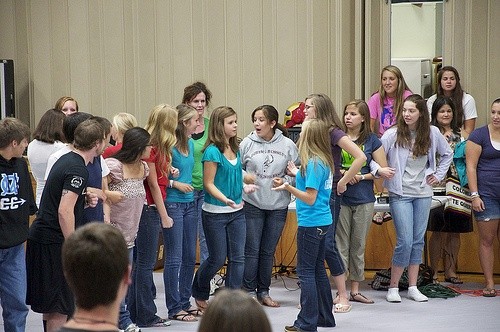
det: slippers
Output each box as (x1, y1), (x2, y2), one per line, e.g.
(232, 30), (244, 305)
(483, 287), (496, 297)
(334, 303), (353, 312)
(432, 278), (442, 285)
(445, 275), (464, 284)
(372, 211), (393, 225)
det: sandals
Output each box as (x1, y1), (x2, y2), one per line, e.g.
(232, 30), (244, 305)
(169, 305), (204, 321)
(349, 292), (374, 304)
(258, 294), (280, 307)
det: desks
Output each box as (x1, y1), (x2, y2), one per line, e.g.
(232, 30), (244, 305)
(287, 195), (453, 275)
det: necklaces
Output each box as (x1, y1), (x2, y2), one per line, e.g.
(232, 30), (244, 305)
(70, 316), (118, 327)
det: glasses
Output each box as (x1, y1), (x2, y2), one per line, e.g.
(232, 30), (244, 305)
(303, 104), (314, 110)
(146, 142), (154, 148)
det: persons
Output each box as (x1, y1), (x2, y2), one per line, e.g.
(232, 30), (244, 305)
(0, 65), (500, 332)
(52, 221), (133, 332)
(197, 288), (274, 332)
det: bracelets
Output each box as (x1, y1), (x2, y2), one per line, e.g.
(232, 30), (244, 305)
(168, 179), (173, 188)
(360, 175), (365, 180)
(471, 191), (480, 199)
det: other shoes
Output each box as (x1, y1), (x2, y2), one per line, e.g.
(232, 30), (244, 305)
(120, 323), (142, 332)
(284, 325), (303, 332)
(149, 318), (172, 327)
(407, 288), (429, 302)
(386, 287), (402, 302)
(209, 274), (223, 295)
